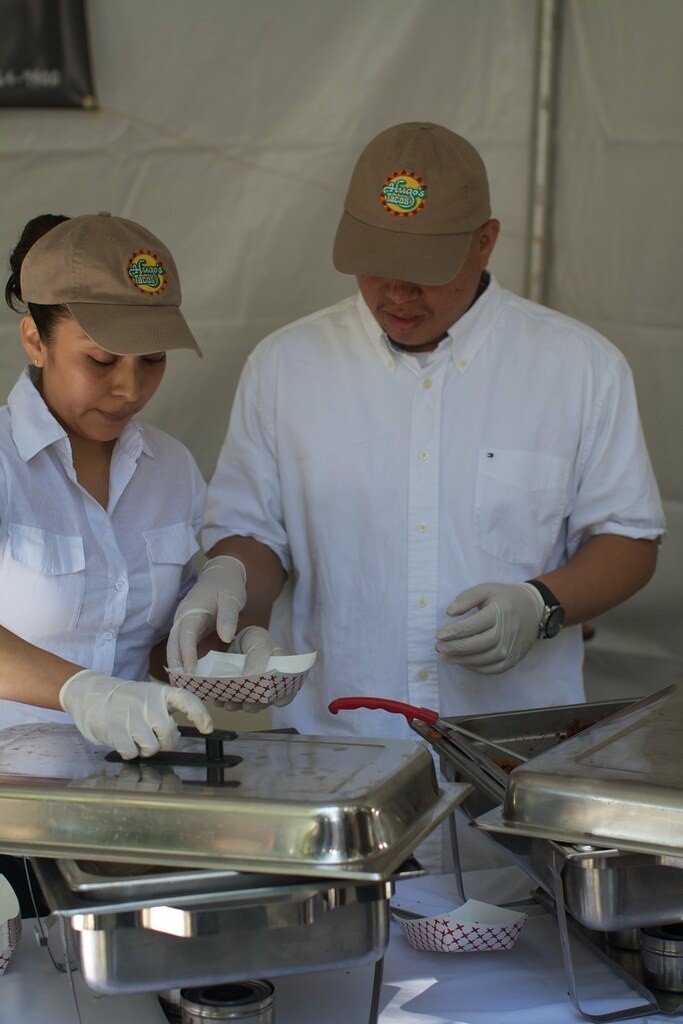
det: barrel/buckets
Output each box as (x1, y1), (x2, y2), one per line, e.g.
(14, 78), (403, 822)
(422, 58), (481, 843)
(639, 929), (682, 994)
(607, 929), (643, 951)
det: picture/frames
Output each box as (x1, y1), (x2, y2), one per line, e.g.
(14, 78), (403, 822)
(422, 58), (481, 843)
(1, 0), (99, 116)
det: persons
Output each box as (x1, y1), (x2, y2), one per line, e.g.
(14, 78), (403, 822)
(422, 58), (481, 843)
(162, 104), (669, 891)
(0, 205), (271, 929)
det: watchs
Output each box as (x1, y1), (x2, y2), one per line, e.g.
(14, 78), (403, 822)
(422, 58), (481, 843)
(522, 575), (565, 642)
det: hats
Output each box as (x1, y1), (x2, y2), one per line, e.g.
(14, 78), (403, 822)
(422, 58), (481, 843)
(332, 123), (492, 286)
(20, 212), (202, 354)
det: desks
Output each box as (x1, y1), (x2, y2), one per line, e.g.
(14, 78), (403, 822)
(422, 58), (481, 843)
(1, 847), (683, 1024)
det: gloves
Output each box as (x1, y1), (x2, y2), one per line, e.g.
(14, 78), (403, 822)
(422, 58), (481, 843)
(216, 626), (297, 714)
(59, 670), (212, 759)
(164, 555), (249, 672)
(435, 581), (550, 677)
